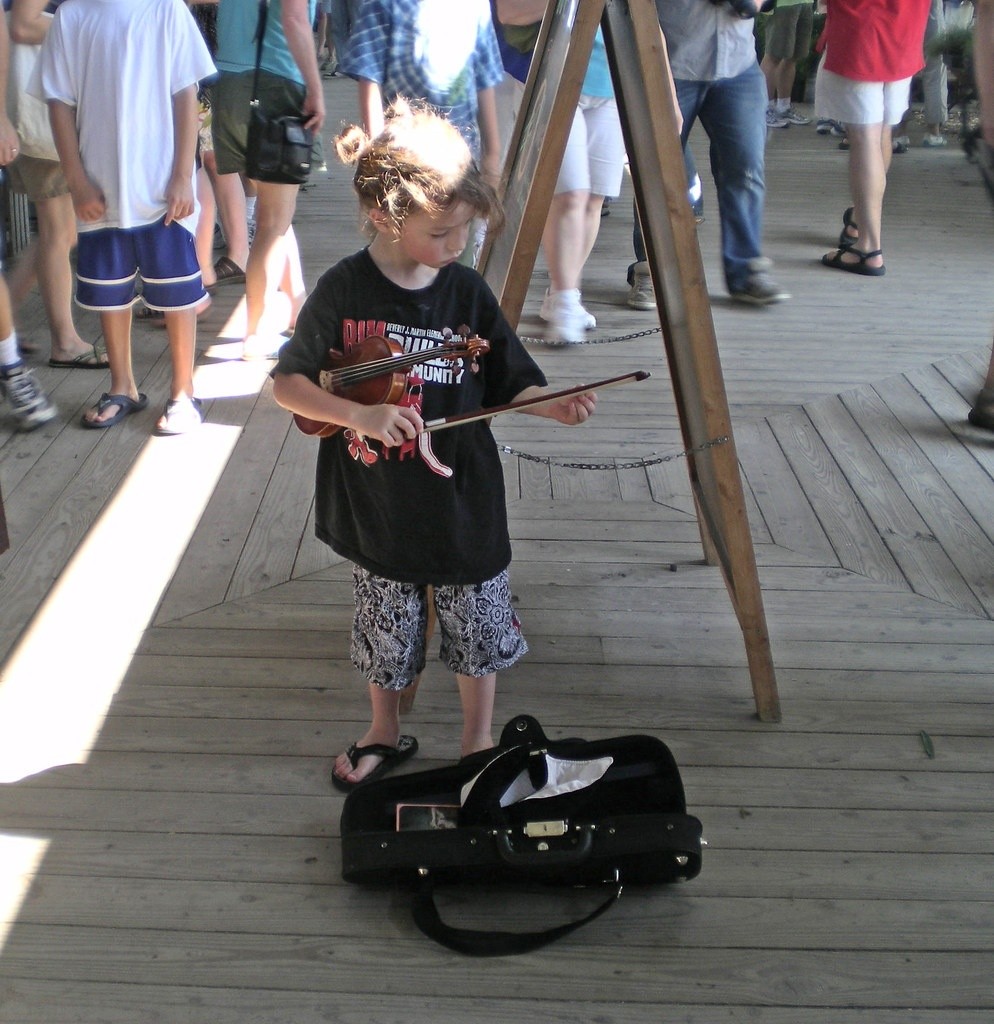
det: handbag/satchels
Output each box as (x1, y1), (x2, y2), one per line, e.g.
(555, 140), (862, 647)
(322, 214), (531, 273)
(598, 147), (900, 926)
(245, 109), (314, 184)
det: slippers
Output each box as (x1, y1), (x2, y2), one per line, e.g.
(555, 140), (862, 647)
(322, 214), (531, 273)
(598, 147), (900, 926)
(212, 255), (246, 286)
(48, 343), (111, 369)
(153, 397), (204, 434)
(331, 735), (418, 792)
(80, 392), (149, 428)
(205, 285), (218, 296)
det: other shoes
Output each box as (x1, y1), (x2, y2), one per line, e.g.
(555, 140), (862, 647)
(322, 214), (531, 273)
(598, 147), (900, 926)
(537, 287), (597, 344)
(317, 50), (347, 79)
(213, 223), (224, 249)
(816, 118), (948, 152)
(968, 391), (994, 430)
(246, 219), (256, 250)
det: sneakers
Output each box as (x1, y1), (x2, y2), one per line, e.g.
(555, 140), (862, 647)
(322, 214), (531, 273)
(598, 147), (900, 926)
(781, 102), (813, 125)
(766, 107), (790, 128)
(628, 261), (657, 309)
(0, 359), (58, 432)
(731, 257), (791, 304)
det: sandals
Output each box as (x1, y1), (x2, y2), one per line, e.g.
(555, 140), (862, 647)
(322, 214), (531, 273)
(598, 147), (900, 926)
(822, 246), (886, 276)
(841, 207), (859, 244)
(136, 306), (165, 319)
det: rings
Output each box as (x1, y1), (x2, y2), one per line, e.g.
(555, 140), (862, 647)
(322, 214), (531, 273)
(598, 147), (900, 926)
(11, 148), (16, 152)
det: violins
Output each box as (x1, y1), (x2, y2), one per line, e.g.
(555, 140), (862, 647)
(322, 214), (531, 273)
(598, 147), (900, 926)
(289, 324), (490, 437)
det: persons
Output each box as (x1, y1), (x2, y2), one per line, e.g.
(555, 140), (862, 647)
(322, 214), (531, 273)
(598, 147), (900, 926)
(1, 0), (218, 433)
(490, 0), (936, 348)
(270, 92), (598, 792)
(184, 0), (506, 363)
(968, 0), (993, 432)
